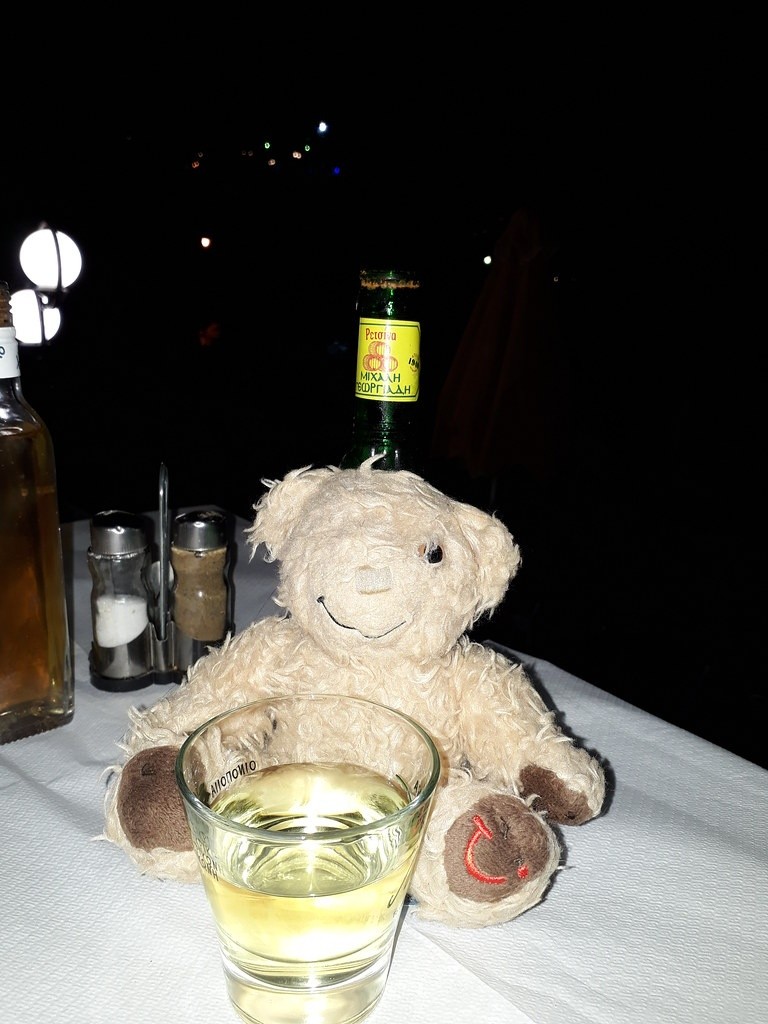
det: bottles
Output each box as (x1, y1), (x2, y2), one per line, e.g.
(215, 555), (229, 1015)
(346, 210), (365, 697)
(169, 504), (232, 671)
(0, 280), (75, 746)
(86, 510), (157, 679)
(335, 266), (463, 503)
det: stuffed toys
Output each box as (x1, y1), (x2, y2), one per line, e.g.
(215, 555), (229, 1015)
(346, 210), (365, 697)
(99, 450), (607, 932)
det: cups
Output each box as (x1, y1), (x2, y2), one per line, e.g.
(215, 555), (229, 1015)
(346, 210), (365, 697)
(174, 694), (441, 1024)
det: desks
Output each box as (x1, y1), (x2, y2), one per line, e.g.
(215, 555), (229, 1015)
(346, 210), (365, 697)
(0, 505), (768, 1024)
